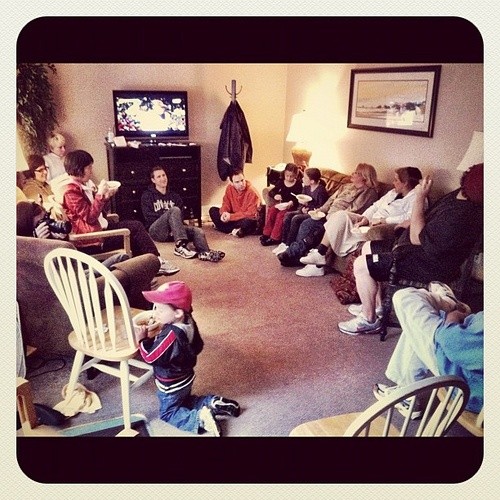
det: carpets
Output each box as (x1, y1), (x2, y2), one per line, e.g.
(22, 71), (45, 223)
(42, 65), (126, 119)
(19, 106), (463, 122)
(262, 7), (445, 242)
(58, 412), (156, 436)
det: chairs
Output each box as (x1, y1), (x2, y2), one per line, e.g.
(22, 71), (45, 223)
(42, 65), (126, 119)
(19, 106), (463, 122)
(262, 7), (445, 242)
(288, 375), (471, 436)
(436, 386), (484, 437)
(16, 186), (134, 261)
(379, 226), (482, 342)
(43, 247), (162, 437)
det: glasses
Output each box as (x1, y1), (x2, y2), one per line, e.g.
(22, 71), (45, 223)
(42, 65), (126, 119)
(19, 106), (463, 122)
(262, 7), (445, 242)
(34, 166), (49, 173)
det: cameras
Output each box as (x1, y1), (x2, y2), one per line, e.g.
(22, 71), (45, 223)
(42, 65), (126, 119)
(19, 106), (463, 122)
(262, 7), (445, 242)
(35, 216), (72, 237)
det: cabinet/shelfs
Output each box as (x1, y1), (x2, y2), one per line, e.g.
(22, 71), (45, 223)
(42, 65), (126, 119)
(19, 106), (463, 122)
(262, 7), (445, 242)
(103, 139), (203, 229)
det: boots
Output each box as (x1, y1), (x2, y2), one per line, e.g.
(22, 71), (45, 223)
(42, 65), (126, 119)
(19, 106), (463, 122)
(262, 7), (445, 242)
(278, 242), (304, 267)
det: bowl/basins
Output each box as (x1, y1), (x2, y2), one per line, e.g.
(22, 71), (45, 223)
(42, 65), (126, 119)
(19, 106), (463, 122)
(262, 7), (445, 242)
(98, 179), (121, 192)
(296, 194), (312, 204)
(308, 210), (326, 220)
(132, 309), (161, 337)
(351, 226), (370, 239)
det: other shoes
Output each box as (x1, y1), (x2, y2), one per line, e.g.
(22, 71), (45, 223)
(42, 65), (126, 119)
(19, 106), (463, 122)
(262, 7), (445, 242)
(272, 242), (290, 256)
(229, 227), (243, 238)
(260, 235), (282, 246)
(211, 224), (217, 229)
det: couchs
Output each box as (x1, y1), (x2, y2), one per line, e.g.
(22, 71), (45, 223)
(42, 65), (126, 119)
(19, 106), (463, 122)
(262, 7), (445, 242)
(16, 234), (161, 382)
(261, 168), (446, 284)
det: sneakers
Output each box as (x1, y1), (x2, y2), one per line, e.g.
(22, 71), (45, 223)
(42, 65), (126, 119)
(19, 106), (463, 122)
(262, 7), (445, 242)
(156, 258), (180, 276)
(427, 281), (471, 314)
(373, 383), (425, 420)
(199, 405), (222, 438)
(198, 249), (225, 262)
(338, 312), (382, 336)
(296, 264), (325, 277)
(300, 248), (326, 265)
(211, 395), (241, 417)
(174, 245), (197, 259)
(347, 304), (384, 319)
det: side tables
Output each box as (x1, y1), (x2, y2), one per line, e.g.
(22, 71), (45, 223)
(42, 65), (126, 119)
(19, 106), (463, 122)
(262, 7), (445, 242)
(267, 163), (291, 187)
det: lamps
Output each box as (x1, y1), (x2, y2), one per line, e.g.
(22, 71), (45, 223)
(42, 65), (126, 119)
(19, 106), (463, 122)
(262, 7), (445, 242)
(285, 113), (318, 167)
(456, 130), (485, 172)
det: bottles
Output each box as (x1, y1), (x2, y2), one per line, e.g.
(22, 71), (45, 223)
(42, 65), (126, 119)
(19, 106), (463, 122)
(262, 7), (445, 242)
(150, 133), (157, 146)
(275, 203), (290, 211)
(108, 128), (114, 143)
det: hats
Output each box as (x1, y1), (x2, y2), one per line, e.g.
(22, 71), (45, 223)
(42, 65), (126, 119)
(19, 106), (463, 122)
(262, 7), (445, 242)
(141, 281), (193, 312)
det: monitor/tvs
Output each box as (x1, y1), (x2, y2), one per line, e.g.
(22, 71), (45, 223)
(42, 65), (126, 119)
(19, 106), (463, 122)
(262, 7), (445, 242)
(112, 89), (191, 145)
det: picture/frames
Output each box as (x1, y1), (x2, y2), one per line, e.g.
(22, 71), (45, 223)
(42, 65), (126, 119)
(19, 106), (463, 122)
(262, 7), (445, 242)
(346, 64), (443, 138)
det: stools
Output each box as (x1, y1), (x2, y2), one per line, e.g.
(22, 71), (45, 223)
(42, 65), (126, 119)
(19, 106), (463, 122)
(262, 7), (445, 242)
(16, 375), (38, 432)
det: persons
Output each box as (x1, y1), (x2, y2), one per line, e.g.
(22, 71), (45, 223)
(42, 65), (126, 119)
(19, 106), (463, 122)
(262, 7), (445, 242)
(338, 163), (483, 336)
(295, 167), (428, 278)
(373, 282), (483, 421)
(260, 163), (303, 246)
(64, 150), (179, 275)
(209, 167), (262, 238)
(277, 163), (379, 267)
(141, 166), (225, 263)
(272, 168), (328, 255)
(22, 154), (70, 221)
(43, 133), (75, 205)
(137, 281), (241, 438)
